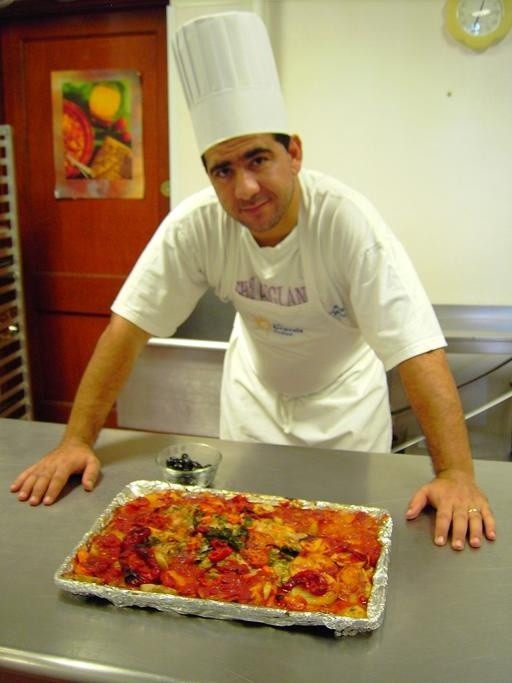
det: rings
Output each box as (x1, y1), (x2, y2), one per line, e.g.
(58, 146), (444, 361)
(468, 508), (480, 513)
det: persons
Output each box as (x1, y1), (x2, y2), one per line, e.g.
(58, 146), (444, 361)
(8, 133), (496, 551)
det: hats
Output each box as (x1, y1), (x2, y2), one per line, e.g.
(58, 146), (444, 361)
(169, 11), (291, 160)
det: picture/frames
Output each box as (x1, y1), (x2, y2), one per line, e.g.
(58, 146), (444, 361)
(48, 68), (145, 198)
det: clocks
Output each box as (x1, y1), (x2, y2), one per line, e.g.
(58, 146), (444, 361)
(442, 1), (511, 53)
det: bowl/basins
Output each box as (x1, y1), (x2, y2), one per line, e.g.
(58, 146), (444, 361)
(63, 80), (126, 180)
(151, 437), (225, 492)
